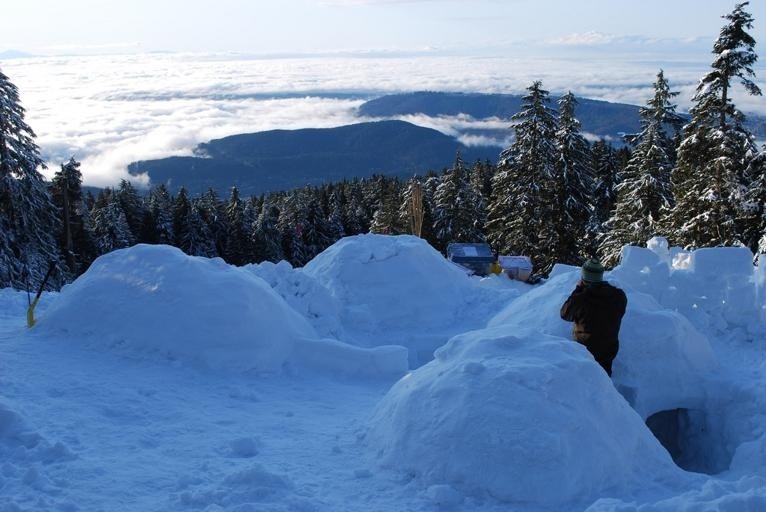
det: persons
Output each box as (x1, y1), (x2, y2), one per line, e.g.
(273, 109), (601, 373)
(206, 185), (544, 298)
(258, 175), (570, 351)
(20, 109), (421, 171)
(559, 257), (628, 378)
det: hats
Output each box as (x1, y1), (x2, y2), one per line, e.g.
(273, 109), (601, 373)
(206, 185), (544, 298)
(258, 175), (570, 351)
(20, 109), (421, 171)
(581, 258), (604, 282)
